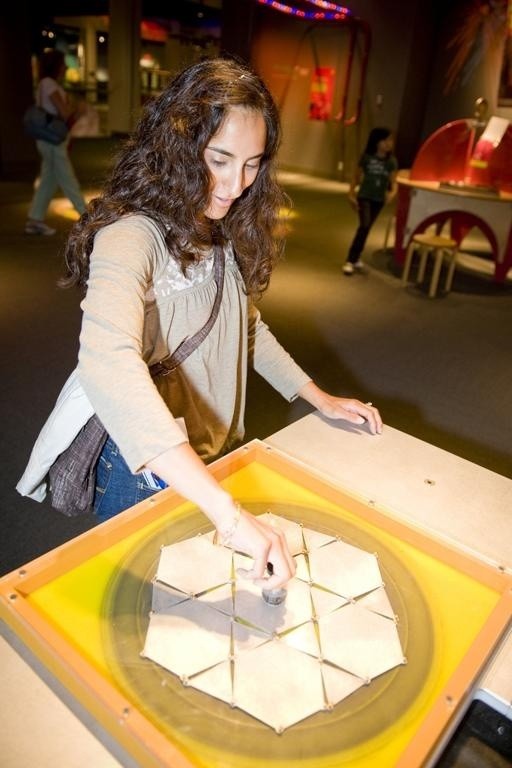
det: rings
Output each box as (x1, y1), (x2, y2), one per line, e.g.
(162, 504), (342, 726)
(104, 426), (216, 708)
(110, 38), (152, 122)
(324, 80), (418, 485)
(365, 401), (373, 406)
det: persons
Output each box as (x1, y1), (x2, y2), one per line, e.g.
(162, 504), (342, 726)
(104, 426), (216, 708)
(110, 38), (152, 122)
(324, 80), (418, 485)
(14, 52), (381, 591)
(22, 53), (95, 236)
(343, 127), (399, 275)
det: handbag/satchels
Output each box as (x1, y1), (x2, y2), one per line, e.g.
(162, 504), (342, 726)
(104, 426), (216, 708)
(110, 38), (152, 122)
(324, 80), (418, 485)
(24, 104), (67, 144)
(48, 413), (108, 517)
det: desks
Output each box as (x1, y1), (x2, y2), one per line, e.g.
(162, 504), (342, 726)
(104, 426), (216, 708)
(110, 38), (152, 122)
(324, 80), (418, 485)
(395, 118), (512, 285)
(0, 407), (512, 768)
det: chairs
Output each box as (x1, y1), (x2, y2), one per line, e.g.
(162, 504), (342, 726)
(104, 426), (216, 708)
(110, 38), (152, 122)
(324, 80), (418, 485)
(383, 169), (411, 252)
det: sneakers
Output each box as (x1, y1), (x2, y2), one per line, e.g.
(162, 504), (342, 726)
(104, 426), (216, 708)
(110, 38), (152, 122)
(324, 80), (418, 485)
(25, 221), (57, 235)
(343, 259), (363, 274)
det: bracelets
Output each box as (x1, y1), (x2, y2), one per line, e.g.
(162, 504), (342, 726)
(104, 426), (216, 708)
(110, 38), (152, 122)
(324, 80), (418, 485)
(209, 500), (240, 551)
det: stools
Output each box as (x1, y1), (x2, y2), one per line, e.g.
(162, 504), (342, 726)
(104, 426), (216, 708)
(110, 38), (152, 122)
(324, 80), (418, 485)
(402, 234), (457, 297)
